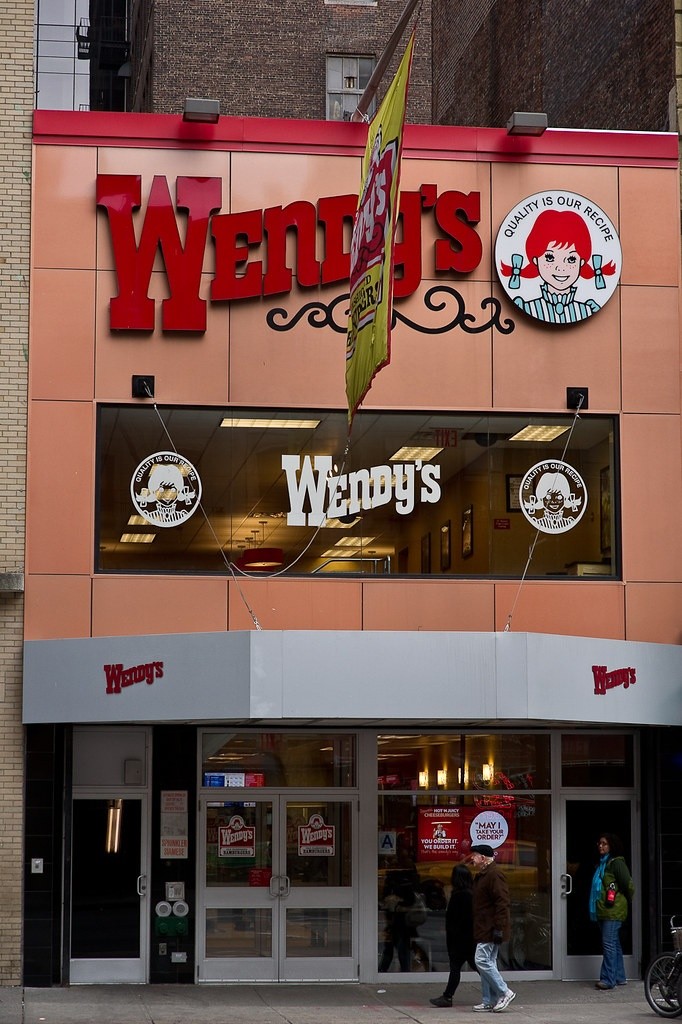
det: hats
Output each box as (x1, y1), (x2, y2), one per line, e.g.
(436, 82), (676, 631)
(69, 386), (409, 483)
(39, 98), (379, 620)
(470, 844), (494, 857)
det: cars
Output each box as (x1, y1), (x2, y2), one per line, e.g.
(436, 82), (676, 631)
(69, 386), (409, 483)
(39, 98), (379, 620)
(206, 916), (312, 958)
(378, 839), (578, 911)
(292, 917), (430, 973)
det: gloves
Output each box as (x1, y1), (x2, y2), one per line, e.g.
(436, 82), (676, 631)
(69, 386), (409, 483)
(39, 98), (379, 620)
(493, 924), (504, 946)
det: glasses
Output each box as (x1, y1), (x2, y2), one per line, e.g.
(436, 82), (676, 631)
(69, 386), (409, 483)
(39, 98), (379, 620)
(597, 842), (608, 846)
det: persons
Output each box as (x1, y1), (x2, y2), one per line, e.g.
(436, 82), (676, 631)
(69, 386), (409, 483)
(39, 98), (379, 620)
(470, 845), (516, 1013)
(589, 833), (636, 989)
(231, 824), (272, 930)
(429, 864), (497, 1007)
(378, 847), (436, 972)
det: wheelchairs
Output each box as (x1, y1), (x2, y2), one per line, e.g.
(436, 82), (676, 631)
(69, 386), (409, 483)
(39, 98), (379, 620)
(644, 915), (682, 1018)
(509, 890), (551, 970)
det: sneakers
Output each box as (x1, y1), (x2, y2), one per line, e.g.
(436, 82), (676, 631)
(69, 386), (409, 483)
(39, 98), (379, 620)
(472, 1003), (495, 1012)
(429, 997), (453, 1007)
(595, 981), (627, 989)
(493, 990), (516, 1012)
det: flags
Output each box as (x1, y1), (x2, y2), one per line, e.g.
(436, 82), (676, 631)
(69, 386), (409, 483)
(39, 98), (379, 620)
(345, 27), (416, 432)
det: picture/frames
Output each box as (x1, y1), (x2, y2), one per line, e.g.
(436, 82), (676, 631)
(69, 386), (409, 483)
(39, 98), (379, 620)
(507, 474), (536, 513)
(599, 465), (611, 554)
(460, 504), (473, 558)
(439, 520), (451, 570)
(420, 532), (431, 573)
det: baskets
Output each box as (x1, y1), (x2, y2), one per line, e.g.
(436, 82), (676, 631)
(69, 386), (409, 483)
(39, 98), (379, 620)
(671, 927), (682, 948)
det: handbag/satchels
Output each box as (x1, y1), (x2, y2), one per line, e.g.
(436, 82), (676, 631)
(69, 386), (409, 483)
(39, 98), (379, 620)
(605, 888), (617, 908)
(409, 893), (427, 925)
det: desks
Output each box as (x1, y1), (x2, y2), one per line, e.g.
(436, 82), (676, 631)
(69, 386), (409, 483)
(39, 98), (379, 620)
(565, 562), (612, 576)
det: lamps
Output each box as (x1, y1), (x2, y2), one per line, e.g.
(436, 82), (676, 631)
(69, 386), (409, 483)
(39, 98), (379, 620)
(507, 112), (547, 137)
(418, 772), (426, 787)
(458, 767), (465, 784)
(184, 97), (219, 123)
(482, 764), (491, 781)
(437, 770), (445, 785)
